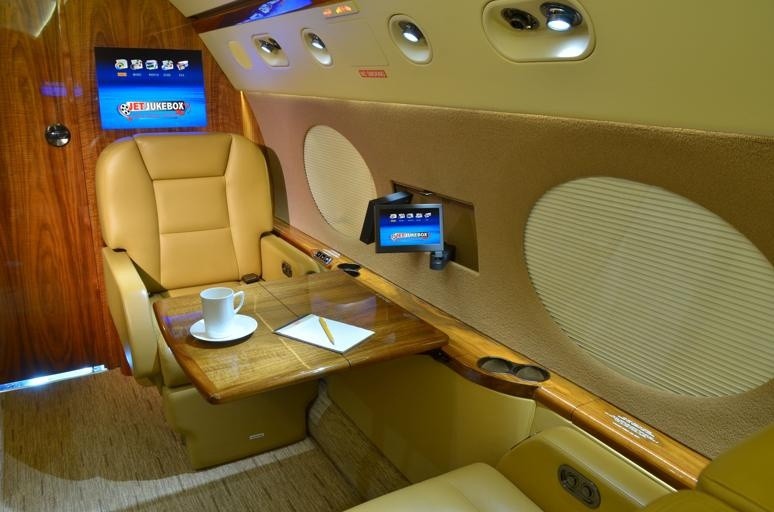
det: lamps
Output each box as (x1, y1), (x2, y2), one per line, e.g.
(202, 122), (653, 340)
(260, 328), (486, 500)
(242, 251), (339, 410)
(401, 23), (421, 43)
(547, 6), (573, 31)
(312, 39), (324, 49)
(260, 41), (273, 53)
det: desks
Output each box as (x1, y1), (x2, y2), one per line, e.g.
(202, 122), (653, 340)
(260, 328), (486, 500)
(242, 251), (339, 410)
(155, 268), (449, 405)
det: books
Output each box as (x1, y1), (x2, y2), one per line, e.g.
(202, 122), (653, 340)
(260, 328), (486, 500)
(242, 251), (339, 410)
(272, 313), (375, 353)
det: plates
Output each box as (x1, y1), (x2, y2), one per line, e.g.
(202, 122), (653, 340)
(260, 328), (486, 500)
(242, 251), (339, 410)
(188, 312), (258, 343)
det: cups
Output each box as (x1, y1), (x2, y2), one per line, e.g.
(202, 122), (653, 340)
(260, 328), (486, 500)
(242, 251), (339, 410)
(198, 286), (244, 340)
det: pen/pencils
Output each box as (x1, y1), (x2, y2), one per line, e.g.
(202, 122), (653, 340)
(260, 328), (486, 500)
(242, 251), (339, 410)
(318, 316), (336, 345)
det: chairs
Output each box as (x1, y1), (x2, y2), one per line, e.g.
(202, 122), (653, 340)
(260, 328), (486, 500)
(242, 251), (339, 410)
(94, 131), (324, 470)
(331, 424), (774, 512)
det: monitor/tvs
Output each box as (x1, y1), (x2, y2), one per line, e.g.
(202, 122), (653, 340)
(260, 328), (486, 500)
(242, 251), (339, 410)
(358, 191), (414, 243)
(372, 203), (445, 255)
(94, 47), (210, 131)
